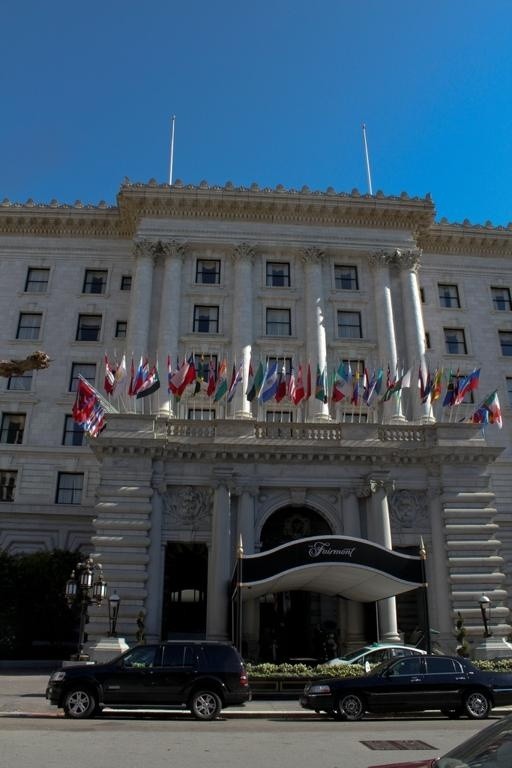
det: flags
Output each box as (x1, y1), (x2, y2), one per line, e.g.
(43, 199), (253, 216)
(471, 387), (503, 431)
(70, 371), (119, 442)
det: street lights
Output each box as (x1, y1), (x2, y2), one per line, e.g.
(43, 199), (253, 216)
(108, 587), (121, 636)
(479, 592), (494, 637)
(63, 556), (108, 662)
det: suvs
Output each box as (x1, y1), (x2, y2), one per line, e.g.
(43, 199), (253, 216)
(42, 639), (252, 720)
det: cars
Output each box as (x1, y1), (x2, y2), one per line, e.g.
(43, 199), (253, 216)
(370, 714), (512, 767)
(321, 641), (441, 668)
(299, 654), (512, 719)
